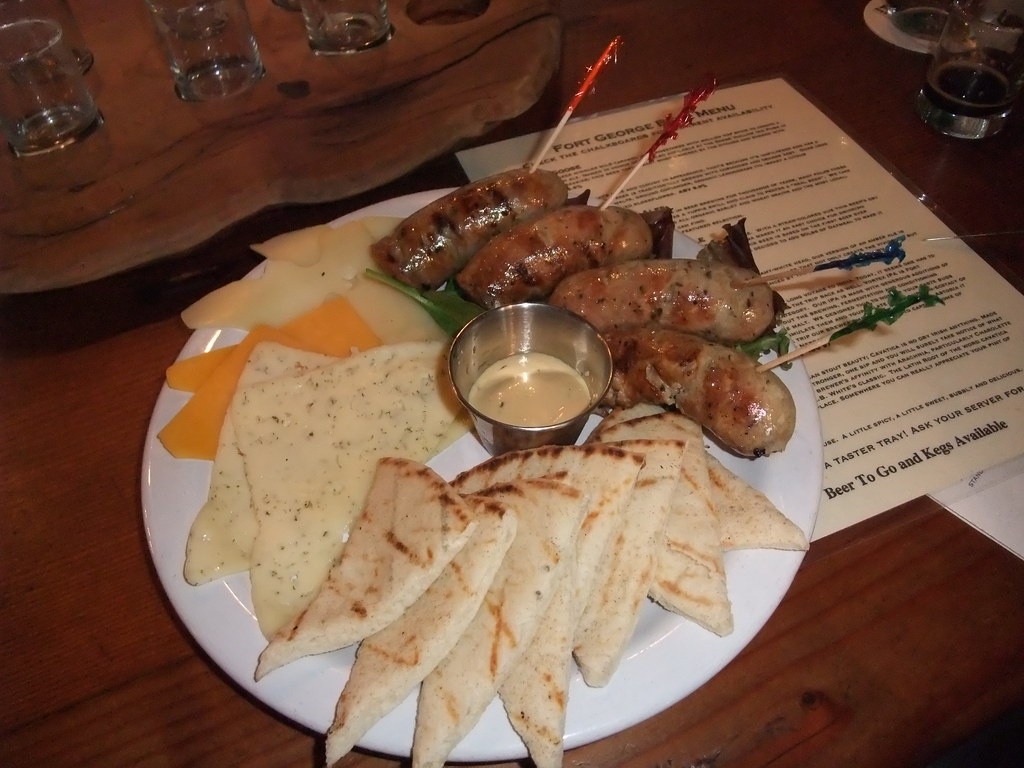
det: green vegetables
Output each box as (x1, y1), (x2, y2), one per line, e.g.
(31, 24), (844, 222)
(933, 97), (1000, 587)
(734, 329), (794, 371)
(365, 270), (481, 337)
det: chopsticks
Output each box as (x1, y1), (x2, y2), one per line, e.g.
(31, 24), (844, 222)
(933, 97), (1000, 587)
(529, 36), (715, 212)
(731, 246), (928, 373)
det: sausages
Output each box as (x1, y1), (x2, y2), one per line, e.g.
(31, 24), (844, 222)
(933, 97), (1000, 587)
(370, 168), (795, 457)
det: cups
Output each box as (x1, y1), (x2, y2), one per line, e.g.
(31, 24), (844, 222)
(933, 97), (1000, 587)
(145, 0), (262, 101)
(300, 0), (392, 57)
(0, 0), (102, 158)
(913, 0), (1024, 139)
(448, 304), (614, 456)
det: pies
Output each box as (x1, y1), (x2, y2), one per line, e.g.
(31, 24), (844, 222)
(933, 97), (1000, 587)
(251, 402), (810, 768)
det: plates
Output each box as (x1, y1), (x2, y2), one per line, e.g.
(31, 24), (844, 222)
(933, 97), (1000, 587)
(142, 185), (825, 762)
(863, 0), (952, 54)
